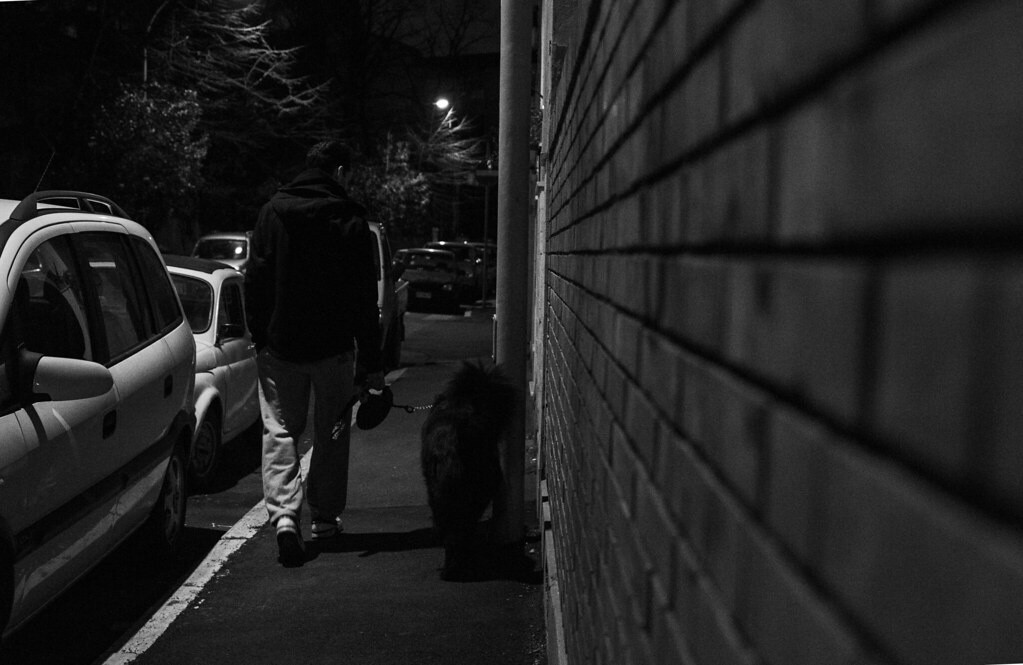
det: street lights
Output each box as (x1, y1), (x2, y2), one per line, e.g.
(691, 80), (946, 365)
(431, 93), (492, 307)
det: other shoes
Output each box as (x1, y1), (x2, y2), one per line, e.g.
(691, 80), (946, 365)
(310, 516), (344, 538)
(276, 515), (306, 563)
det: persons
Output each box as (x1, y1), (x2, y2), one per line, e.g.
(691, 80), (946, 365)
(244, 138), (384, 555)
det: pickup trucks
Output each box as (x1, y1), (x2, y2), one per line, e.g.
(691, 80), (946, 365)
(363, 219), (411, 372)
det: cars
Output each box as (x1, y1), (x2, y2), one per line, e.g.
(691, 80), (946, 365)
(83, 226), (261, 486)
(395, 240), (497, 311)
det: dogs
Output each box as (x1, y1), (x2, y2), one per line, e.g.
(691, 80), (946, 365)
(421, 348), (523, 575)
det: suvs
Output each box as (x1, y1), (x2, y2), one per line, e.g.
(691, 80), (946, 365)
(0, 190), (199, 661)
